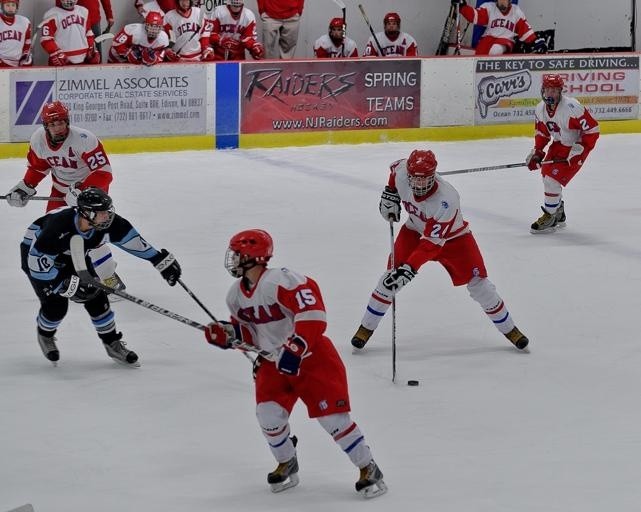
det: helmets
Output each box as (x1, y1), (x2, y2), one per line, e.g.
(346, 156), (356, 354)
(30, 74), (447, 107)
(384, 13), (400, 42)
(0, 0), (19, 17)
(541, 73), (563, 106)
(42, 101), (69, 143)
(330, 18), (346, 46)
(77, 188), (115, 229)
(225, 229), (273, 277)
(145, 12), (163, 37)
(227, 0), (243, 19)
(407, 150), (437, 196)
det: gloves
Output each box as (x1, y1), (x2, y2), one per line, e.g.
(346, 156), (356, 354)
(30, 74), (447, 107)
(205, 320), (243, 348)
(6, 179), (36, 207)
(567, 142), (589, 168)
(86, 48), (100, 64)
(125, 36), (265, 66)
(526, 149), (545, 170)
(60, 275), (101, 302)
(47, 48), (68, 65)
(380, 187), (401, 222)
(150, 249), (181, 286)
(275, 334), (313, 376)
(377, 264), (417, 294)
(18, 52), (34, 67)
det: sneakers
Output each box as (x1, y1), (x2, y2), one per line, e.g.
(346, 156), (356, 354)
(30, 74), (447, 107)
(351, 325), (373, 348)
(356, 459), (382, 490)
(268, 456), (299, 483)
(38, 326), (60, 361)
(505, 327), (528, 349)
(531, 201), (566, 230)
(99, 329), (138, 363)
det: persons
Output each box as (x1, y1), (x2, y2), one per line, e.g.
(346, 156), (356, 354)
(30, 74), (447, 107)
(351, 147), (530, 349)
(6, 100), (127, 297)
(206, 228), (386, 493)
(525, 74), (601, 231)
(18, 186), (184, 364)
